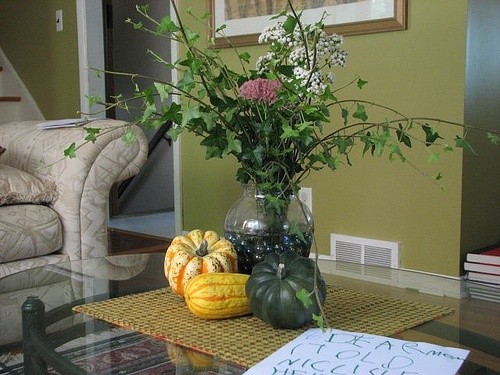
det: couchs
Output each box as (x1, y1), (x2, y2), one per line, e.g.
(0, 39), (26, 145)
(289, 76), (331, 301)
(0, 47), (149, 346)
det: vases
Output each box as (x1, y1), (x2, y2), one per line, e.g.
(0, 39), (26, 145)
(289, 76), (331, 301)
(223, 182), (314, 275)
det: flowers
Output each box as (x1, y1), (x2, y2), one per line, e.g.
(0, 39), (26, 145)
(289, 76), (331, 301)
(36, 0), (500, 334)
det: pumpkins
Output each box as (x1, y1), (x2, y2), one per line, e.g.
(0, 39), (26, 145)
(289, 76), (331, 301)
(246, 252), (327, 328)
(185, 273), (250, 318)
(163, 229), (239, 300)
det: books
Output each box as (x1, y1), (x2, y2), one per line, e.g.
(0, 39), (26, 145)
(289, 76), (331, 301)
(463, 247), (500, 285)
(41, 117), (100, 130)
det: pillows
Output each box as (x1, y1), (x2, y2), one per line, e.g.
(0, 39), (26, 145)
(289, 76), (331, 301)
(0, 163), (57, 208)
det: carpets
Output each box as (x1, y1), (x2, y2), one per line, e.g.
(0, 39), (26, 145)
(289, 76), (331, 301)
(0, 326), (176, 375)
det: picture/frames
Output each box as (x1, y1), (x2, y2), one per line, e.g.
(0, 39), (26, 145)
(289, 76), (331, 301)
(205, 0), (408, 50)
